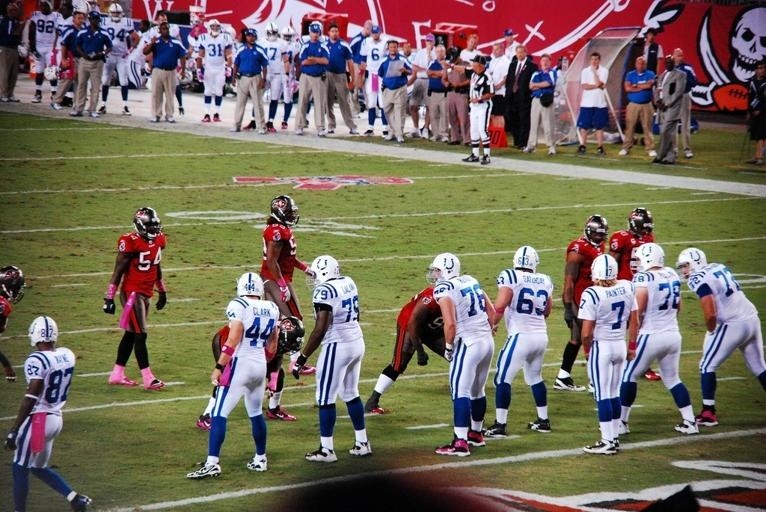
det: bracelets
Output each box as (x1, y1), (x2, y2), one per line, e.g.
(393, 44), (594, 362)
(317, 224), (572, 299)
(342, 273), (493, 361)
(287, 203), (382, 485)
(445, 341), (455, 351)
(628, 340), (638, 350)
(296, 354), (307, 367)
(298, 262), (307, 271)
(105, 283), (118, 299)
(582, 344), (593, 353)
(155, 277), (167, 292)
(215, 363), (224, 371)
(277, 276), (286, 287)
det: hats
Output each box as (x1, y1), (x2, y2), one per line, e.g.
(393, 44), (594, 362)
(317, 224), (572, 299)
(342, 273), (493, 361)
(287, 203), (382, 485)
(445, 48), (458, 64)
(504, 29), (513, 36)
(425, 34), (435, 41)
(469, 56), (486, 65)
(371, 26), (380, 33)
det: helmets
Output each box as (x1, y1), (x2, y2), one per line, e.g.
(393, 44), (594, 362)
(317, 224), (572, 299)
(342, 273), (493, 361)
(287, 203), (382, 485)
(279, 316), (305, 356)
(270, 195), (299, 226)
(44, 65), (58, 80)
(133, 207), (162, 242)
(77, 2), (124, 23)
(18, 45), (28, 58)
(28, 316), (59, 346)
(306, 255), (339, 292)
(0, 266), (27, 305)
(237, 272), (265, 300)
(426, 253), (461, 285)
(207, 19), (323, 42)
(584, 207), (707, 282)
(513, 246), (540, 273)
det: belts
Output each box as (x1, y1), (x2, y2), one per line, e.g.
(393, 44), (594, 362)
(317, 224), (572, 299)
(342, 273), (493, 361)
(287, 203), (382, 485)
(303, 72), (320, 77)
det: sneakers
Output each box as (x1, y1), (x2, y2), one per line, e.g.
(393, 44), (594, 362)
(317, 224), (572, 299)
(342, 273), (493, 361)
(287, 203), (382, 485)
(247, 456), (268, 472)
(644, 369), (661, 381)
(305, 443), (338, 463)
(553, 377), (595, 393)
(528, 414), (552, 433)
(522, 145), (693, 164)
(1, 95), (221, 123)
(481, 155), (490, 164)
(370, 407), (384, 414)
(230, 121), (472, 146)
(582, 420), (631, 455)
(288, 360), (316, 375)
(187, 460), (222, 479)
(434, 420), (508, 457)
(463, 153), (479, 162)
(674, 411), (719, 434)
(348, 439), (372, 457)
(71, 494), (92, 512)
(743, 158), (764, 166)
(266, 406), (296, 421)
(196, 414), (212, 431)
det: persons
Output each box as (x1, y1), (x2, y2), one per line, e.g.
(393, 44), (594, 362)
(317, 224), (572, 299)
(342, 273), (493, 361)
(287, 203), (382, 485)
(102, 204), (170, 389)
(261, 192), (317, 375)
(523, 55), (557, 153)
(413, 29), (538, 164)
(578, 253), (641, 456)
(196, 19), (413, 143)
(553, 214), (610, 396)
(0, 0), (189, 124)
(609, 206), (661, 383)
(3, 313), (96, 511)
(195, 314), (304, 432)
(292, 255), (372, 464)
(0, 263), (26, 386)
(187, 270), (281, 477)
(364, 285), (455, 417)
(746, 61), (766, 164)
(482, 244), (553, 437)
(577, 52), (609, 155)
(618, 29), (697, 157)
(674, 247), (766, 429)
(423, 250), (497, 457)
(622, 240), (700, 436)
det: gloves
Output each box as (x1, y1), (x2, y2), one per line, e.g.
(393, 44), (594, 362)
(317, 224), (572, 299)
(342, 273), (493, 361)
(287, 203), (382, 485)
(266, 371), (279, 397)
(224, 67), (232, 77)
(291, 79), (299, 93)
(154, 290), (166, 310)
(293, 354), (306, 379)
(277, 286), (291, 304)
(197, 68), (203, 82)
(444, 343), (454, 362)
(4, 432), (17, 450)
(564, 302), (573, 327)
(304, 267), (314, 277)
(416, 343), (428, 365)
(102, 298), (115, 314)
(144, 60), (151, 74)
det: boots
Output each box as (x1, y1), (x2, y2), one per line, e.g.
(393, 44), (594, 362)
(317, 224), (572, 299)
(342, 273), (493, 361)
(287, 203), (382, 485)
(141, 367), (164, 390)
(108, 364), (137, 386)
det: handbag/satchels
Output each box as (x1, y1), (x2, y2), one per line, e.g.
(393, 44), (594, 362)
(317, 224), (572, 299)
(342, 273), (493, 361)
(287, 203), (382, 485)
(541, 92), (553, 107)
(669, 82), (690, 95)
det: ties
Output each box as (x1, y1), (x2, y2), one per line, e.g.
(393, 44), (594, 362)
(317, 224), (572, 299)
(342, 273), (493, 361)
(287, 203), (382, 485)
(512, 64), (521, 93)
